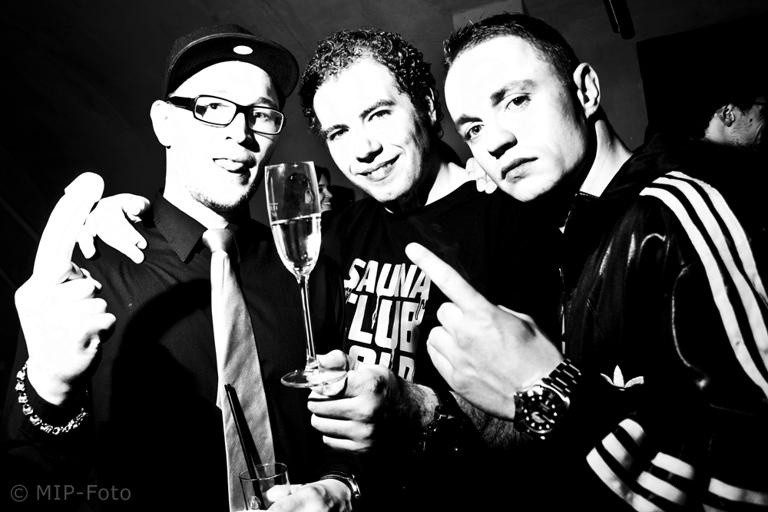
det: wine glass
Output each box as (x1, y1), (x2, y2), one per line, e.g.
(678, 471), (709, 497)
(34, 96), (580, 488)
(264, 159), (348, 389)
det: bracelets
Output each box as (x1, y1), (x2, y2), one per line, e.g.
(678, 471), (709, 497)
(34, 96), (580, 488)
(417, 385), (464, 456)
(320, 471), (362, 511)
(14, 359), (89, 437)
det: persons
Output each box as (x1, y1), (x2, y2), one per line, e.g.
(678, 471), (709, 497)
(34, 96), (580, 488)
(73, 28), (545, 511)
(282, 173), (315, 212)
(312, 167), (334, 211)
(405, 12), (767, 511)
(675, 77), (767, 291)
(1, 23), (364, 512)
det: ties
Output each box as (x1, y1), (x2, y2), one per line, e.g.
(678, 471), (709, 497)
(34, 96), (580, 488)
(202, 229), (276, 511)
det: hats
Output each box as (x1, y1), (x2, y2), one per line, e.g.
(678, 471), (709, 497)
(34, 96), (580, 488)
(165, 23), (299, 111)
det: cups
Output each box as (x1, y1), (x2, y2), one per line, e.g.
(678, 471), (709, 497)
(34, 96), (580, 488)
(239, 464), (290, 510)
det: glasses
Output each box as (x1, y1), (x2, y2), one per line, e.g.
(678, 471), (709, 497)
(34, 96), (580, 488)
(166, 96), (286, 135)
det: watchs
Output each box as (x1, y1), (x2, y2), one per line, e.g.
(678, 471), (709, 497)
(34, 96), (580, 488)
(513, 357), (584, 442)
